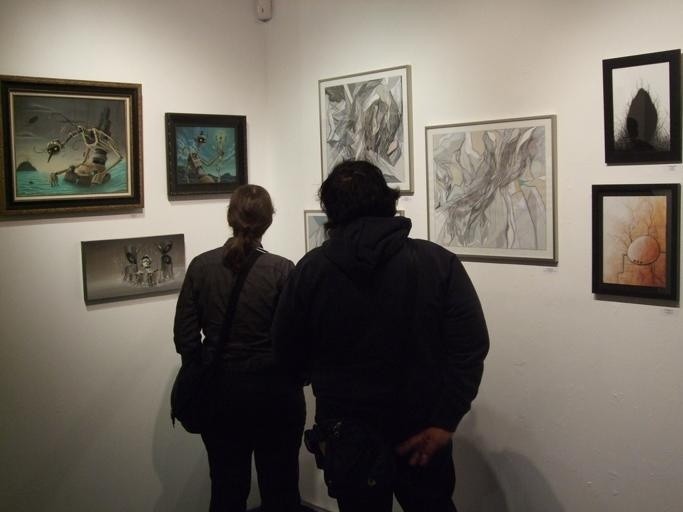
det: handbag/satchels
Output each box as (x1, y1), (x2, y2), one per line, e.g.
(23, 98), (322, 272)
(170, 362), (222, 432)
(303, 419), (391, 497)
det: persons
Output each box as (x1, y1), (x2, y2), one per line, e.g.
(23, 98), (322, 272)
(268, 162), (489, 512)
(169, 184), (306, 512)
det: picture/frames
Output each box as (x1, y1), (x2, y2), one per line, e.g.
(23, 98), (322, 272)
(163, 112), (248, 197)
(424, 113), (558, 267)
(304, 209), (405, 258)
(592, 183), (681, 305)
(0, 74), (145, 220)
(317, 65), (414, 195)
(602, 48), (682, 165)
